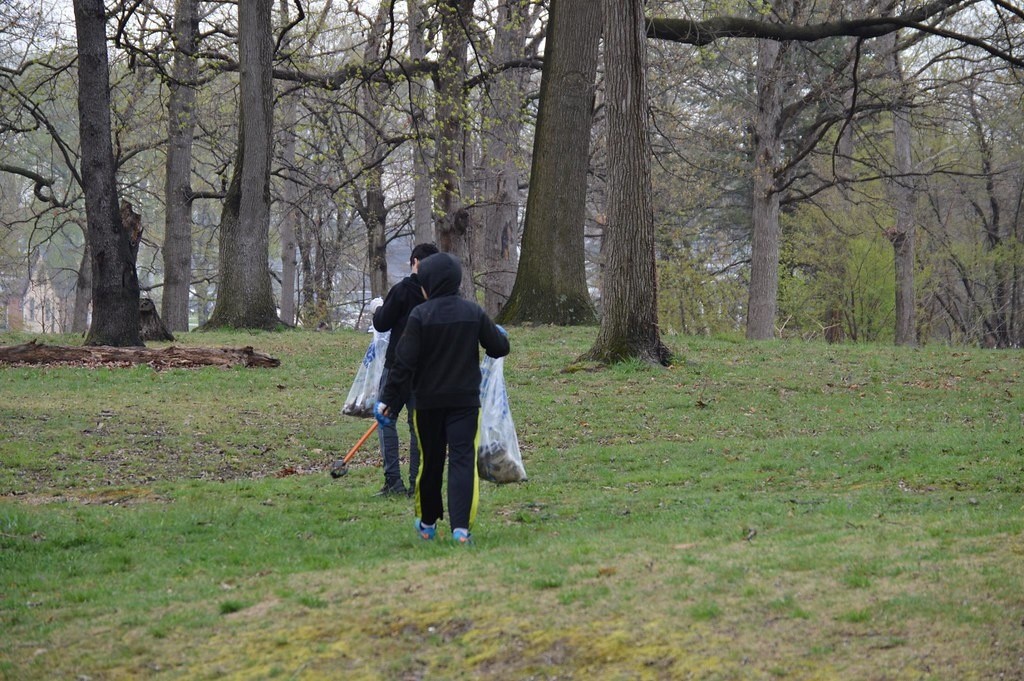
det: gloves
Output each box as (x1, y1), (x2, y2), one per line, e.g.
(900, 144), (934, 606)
(373, 401), (391, 428)
(370, 297), (384, 314)
(496, 324), (508, 336)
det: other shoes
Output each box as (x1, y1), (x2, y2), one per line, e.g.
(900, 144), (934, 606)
(373, 479), (408, 497)
(453, 530), (474, 545)
(408, 486), (414, 496)
(414, 518), (437, 541)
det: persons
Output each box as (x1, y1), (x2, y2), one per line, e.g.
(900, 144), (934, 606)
(372, 252), (509, 544)
(365, 243), (463, 498)
